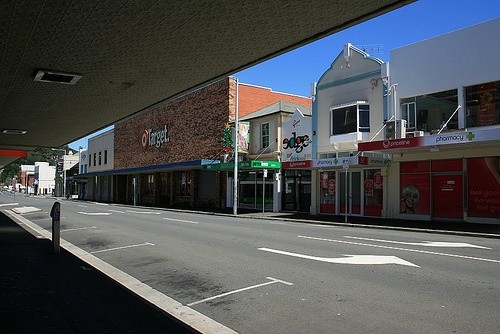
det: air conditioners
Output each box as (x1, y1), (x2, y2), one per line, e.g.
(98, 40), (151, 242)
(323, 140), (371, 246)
(384, 119), (406, 139)
(413, 130), (425, 138)
